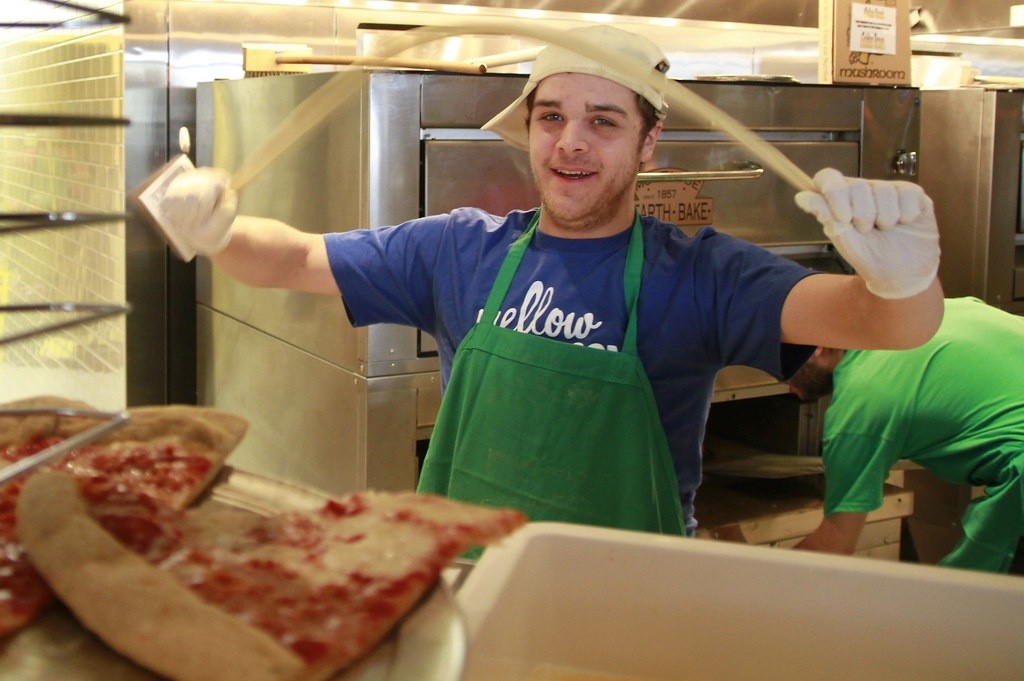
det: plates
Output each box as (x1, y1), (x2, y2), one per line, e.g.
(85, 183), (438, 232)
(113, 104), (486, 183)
(0, 463), (467, 681)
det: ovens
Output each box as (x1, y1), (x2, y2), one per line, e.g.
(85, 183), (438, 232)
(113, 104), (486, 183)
(197, 70), (922, 562)
(920, 88), (1024, 317)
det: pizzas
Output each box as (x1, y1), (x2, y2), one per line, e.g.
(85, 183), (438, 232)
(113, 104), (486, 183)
(17, 471), (527, 681)
(0, 407), (248, 641)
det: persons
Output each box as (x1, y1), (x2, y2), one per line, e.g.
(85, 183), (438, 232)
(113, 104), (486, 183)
(164, 26), (945, 586)
(758, 256), (1024, 580)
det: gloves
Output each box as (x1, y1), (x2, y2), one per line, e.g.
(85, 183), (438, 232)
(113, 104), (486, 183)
(793, 168), (940, 299)
(161, 168), (239, 256)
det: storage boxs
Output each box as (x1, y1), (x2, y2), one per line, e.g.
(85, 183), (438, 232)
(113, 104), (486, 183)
(451, 516), (1024, 681)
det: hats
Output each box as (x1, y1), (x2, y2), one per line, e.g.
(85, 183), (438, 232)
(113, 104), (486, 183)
(480, 25), (671, 152)
